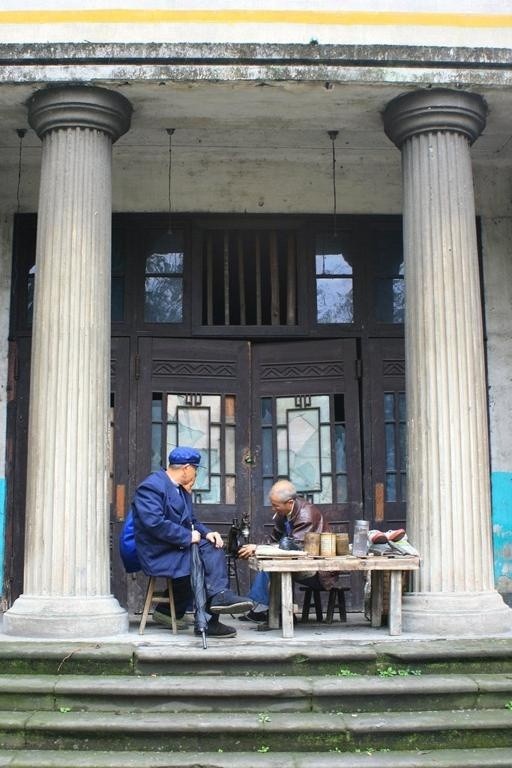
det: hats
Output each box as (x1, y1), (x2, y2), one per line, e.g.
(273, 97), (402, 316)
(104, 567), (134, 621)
(168, 446), (209, 470)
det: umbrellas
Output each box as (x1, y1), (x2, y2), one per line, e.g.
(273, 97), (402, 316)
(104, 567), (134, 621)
(191, 524), (213, 648)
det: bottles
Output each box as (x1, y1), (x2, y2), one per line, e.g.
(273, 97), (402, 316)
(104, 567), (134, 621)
(303, 520), (370, 558)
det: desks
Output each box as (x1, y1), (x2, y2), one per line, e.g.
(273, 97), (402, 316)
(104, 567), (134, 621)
(246, 555), (420, 639)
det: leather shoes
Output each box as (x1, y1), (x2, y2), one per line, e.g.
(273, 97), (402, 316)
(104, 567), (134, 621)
(255, 611), (300, 632)
(237, 608), (270, 622)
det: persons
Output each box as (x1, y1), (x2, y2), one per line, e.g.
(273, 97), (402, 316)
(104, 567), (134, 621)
(239, 478), (338, 624)
(131, 446), (254, 637)
(119, 468), (197, 629)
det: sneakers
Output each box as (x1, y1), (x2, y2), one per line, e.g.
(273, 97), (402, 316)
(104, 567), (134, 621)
(177, 612), (196, 627)
(210, 588), (256, 617)
(191, 616), (238, 639)
(383, 527), (406, 543)
(152, 608), (190, 631)
(367, 527), (388, 545)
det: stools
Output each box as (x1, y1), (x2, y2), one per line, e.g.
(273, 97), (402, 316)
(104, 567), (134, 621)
(138, 575), (178, 636)
(299, 586), (350, 624)
